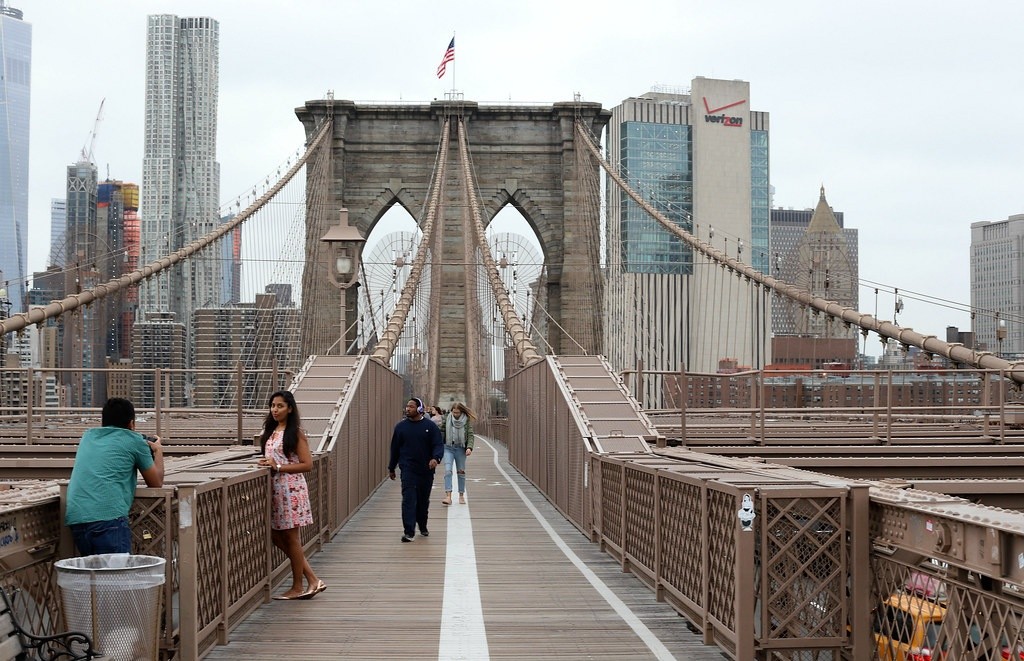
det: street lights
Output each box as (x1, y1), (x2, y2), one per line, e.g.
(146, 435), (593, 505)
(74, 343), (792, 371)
(319, 207), (367, 355)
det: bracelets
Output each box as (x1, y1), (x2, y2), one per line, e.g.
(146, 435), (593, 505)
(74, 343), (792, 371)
(276, 463), (281, 473)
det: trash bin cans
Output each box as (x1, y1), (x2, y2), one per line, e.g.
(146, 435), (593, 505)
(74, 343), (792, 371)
(54, 552), (166, 660)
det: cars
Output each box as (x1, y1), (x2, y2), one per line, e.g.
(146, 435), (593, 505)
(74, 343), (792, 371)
(900, 571), (946, 605)
(968, 619), (1024, 661)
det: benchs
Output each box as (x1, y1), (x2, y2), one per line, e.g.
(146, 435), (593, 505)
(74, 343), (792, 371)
(0, 585), (101, 661)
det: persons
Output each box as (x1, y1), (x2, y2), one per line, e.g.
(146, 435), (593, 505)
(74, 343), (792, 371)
(388, 397), (444, 542)
(64, 398), (165, 555)
(424, 405), (450, 445)
(442, 403), (477, 505)
(259, 390), (327, 600)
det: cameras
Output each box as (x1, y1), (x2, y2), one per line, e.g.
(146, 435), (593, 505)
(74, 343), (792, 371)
(141, 434), (157, 460)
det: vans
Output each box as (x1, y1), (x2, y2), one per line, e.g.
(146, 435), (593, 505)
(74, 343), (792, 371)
(842, 593), (949, 661)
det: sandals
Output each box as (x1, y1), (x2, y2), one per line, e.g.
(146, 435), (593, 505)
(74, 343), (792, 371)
(297, 580), (326, 599)
(273, 591), (305, 600)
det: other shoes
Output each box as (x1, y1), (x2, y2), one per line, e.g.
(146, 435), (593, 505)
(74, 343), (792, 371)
(401, 535), (415, 542)
(459, 496), (466, 504)
(442, 498), (451, 504)
(419, 526), (428, 537)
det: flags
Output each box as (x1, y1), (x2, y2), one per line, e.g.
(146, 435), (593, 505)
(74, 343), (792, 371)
(437, 36), (455, 79)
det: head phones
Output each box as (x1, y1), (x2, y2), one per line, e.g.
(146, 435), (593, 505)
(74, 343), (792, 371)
(415, 398), (423, 414)
